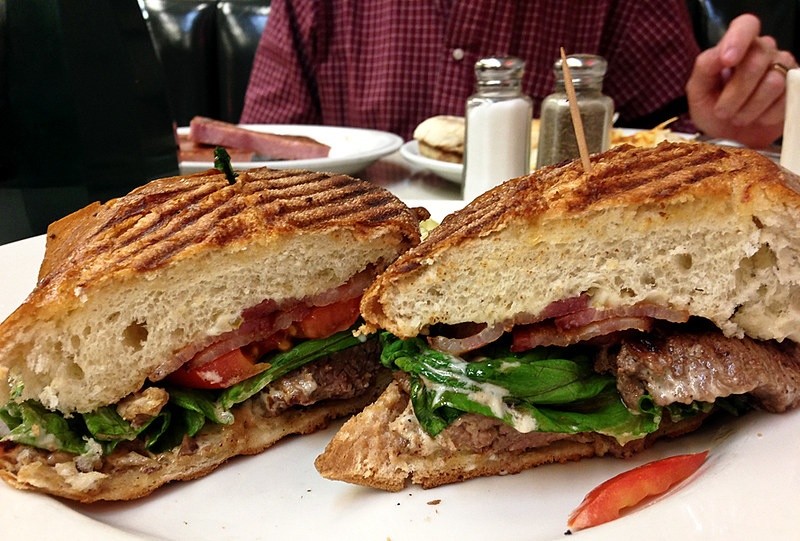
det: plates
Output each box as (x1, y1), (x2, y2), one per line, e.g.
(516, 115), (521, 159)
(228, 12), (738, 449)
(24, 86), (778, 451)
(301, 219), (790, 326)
(174, 123), (402, 183)
(0, 199), (799, 538)
(398, 136), (566, 185)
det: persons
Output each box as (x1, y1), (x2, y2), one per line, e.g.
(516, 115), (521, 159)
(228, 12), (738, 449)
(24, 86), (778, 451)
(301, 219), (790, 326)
(239, 0), (800, 149)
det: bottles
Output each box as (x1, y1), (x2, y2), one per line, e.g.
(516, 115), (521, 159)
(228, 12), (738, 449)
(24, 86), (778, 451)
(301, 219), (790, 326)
(538, 56), (615, 173)
(458, 57), (533, 209)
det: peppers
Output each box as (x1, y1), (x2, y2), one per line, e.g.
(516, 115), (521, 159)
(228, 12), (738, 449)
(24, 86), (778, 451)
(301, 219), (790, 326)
(565, 451), (708, 528)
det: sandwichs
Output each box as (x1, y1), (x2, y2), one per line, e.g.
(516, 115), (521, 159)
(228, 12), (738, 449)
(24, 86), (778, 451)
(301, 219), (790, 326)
(0, 163), (440, 502)
(313, 139), (800, 490)
(413, 115), (465, 163)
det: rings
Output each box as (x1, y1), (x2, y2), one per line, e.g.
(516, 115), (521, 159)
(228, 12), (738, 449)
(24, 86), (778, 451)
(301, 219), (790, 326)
(771, 62), (789, 75)
